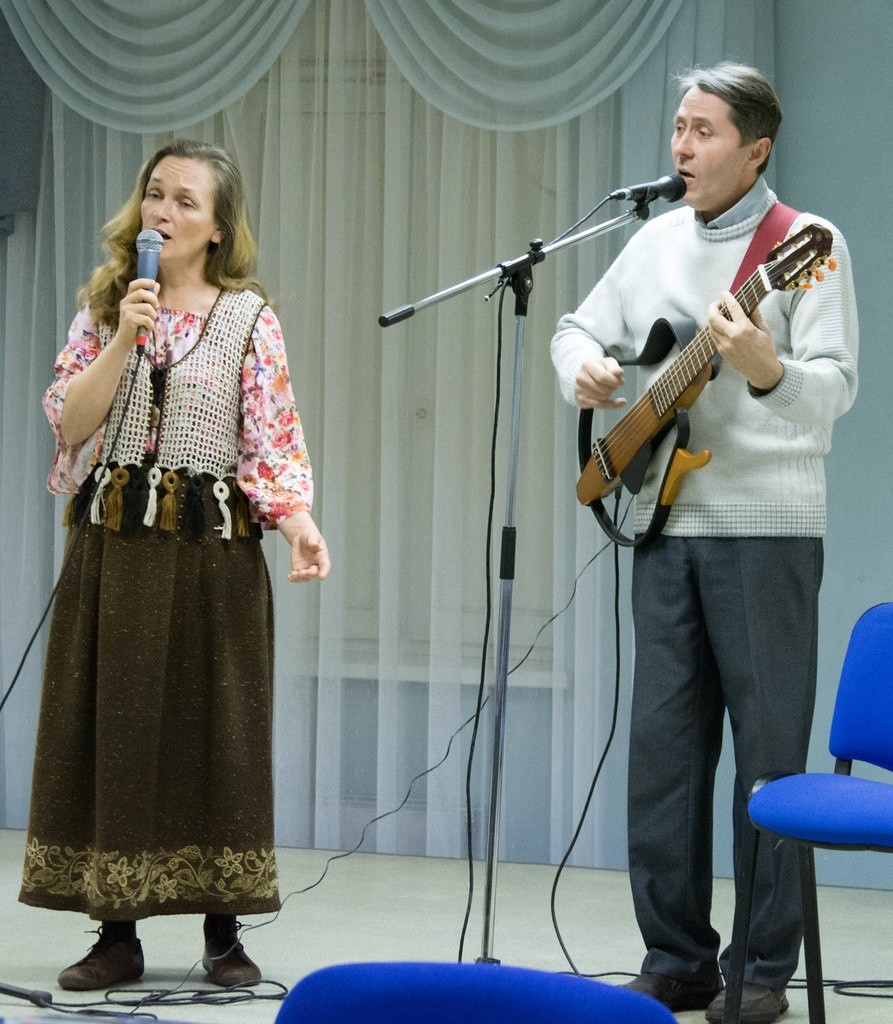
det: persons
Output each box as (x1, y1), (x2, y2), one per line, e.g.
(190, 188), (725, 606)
(547, 64), (861, 1024)
(14, 139), (332, 991)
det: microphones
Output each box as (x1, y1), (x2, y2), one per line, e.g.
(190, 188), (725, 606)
(135, 229), (164, 356)
(608, 172), (687, 203)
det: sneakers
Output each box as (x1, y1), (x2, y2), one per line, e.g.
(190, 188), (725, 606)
(705, 979), (789, 1024)
(623, 973), (720, 1014)
(201, 925), (262, 987)
(57, 939), (146, 991)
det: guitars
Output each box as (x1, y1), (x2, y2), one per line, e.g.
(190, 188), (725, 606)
(575, 222), (837, 546)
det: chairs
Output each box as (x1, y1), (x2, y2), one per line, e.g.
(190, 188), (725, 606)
(719, 602), (893, 1024)
